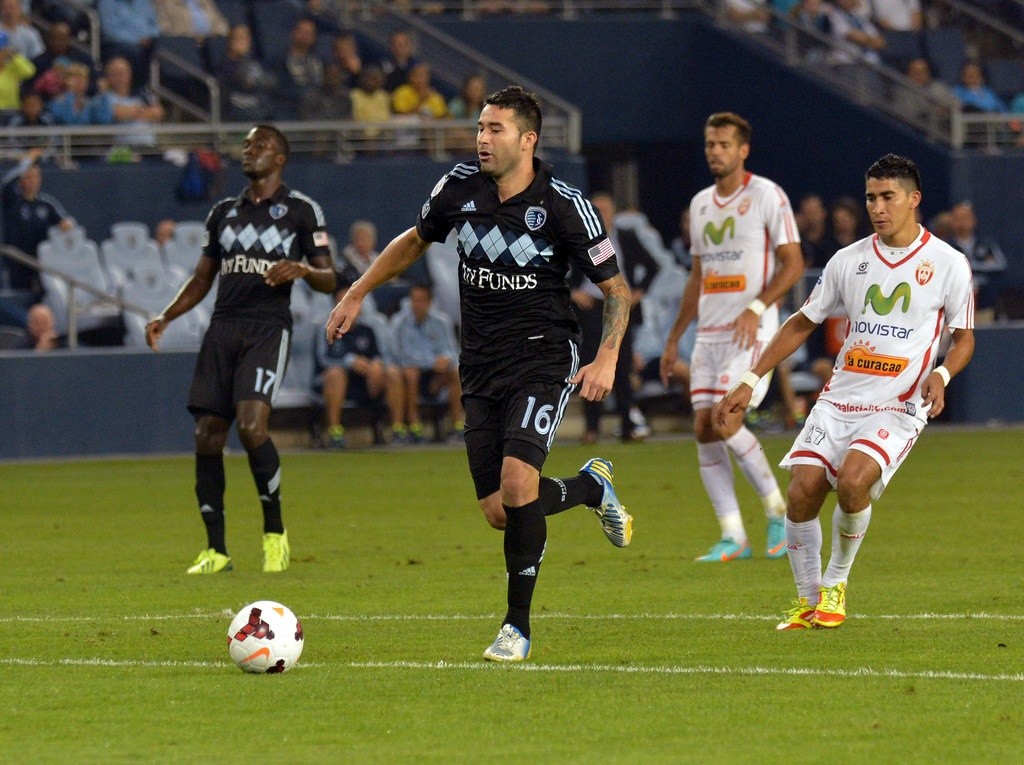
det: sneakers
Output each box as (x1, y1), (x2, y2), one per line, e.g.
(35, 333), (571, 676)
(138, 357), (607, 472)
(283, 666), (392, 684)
(766, 518), (788, 558)
(812, 582), (847, 628)
(262, 528), (291, 573)
(187, 548), (233, 575)
(776, 595), (819, 631)
(482, 622), (531, 662)
(579, 457), (633, 549)
(695, 536), (752, 562)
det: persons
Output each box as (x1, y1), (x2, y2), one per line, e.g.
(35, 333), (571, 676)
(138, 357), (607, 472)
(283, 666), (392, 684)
(716, 154), (974, 629)
(711, 0), (1024, 148)
(312, 222), (465, 446)
(0, 0), (166, 160)
(660, 112), (804, 562)
(569, 192), (690, 443)
(744, 293), (849, 434)
(329, 87), (632, 660)
(147, 124), (338, 575)
(156, 0), (489, 156)
(0, 148), (71, 351)
(791, 196), (1024, 322)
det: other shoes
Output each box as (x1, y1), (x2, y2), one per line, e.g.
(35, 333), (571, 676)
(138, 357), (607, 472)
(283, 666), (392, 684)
(325, 423), (466, 448)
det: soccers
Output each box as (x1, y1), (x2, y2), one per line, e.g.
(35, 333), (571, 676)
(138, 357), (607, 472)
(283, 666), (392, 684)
(225, 599), (304, 674)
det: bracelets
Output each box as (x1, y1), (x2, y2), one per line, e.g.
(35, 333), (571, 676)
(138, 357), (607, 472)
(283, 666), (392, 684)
(748, 300), (765, 315)
(740, 371), (760, 388)
(933, 366), (951, 388)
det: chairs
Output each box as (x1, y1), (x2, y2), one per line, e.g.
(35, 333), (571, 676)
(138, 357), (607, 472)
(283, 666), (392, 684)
(0, 0), (822, 400)
